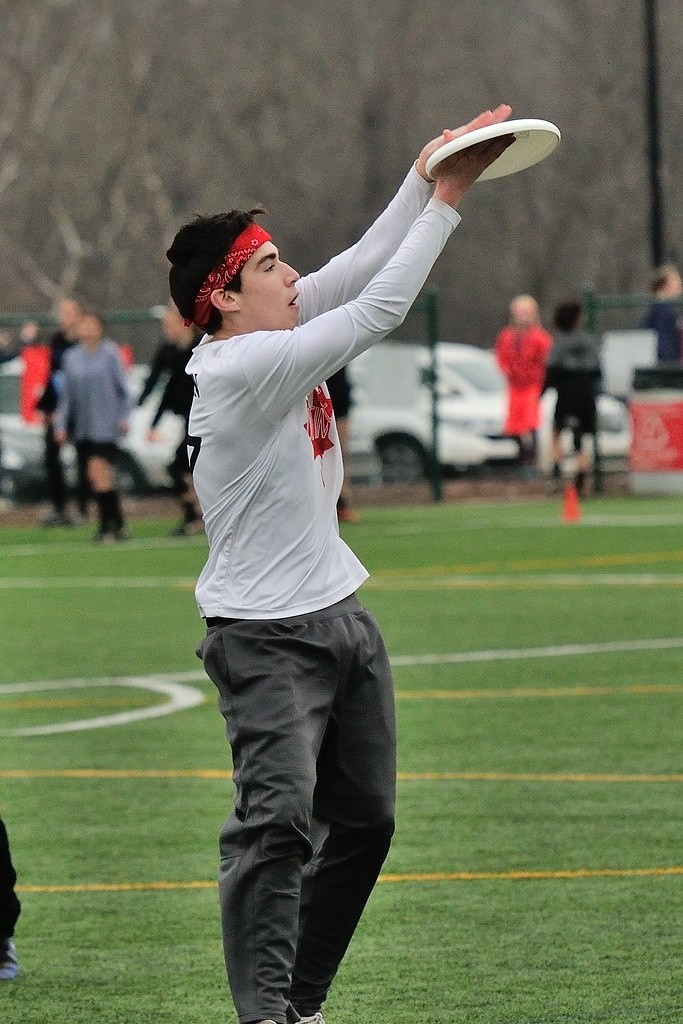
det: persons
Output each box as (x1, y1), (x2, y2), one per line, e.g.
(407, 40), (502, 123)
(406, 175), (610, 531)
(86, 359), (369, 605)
(325, 365), (362, 522)
(0, 816), (21, 979)
(1, 298), (130, 543)
(165, 106), (515, 1024)
(498, 296), (602, 494)
(638, 266), (683, 362)
(135, 299), (203, 536)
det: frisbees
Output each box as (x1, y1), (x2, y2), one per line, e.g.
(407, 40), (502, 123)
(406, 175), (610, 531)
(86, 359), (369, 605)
(426, 117), (560, 180)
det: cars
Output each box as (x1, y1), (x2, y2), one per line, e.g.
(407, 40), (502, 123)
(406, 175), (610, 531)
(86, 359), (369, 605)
(346, 346), (519, 483)
(118, 383), (187, 493)
(2, 359), (80, 500)
(431, 339), (633, 464)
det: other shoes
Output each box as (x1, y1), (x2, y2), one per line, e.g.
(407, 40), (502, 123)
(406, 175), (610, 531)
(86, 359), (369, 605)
(37, 512), (71, 526)
(337, 508), (361, 524)
(88, 526), (134, 547)
(168, 519), (205, 536)
(73, 514), (93, 527)
(511, 431), (541, 465)
(590, 471), (606, 494)
(549, 462), (564, 482)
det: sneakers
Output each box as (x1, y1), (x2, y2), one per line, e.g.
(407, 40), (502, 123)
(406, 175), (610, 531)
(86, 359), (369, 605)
(0, 938), (20, 980)
(252, 1011), (325, 1024)
